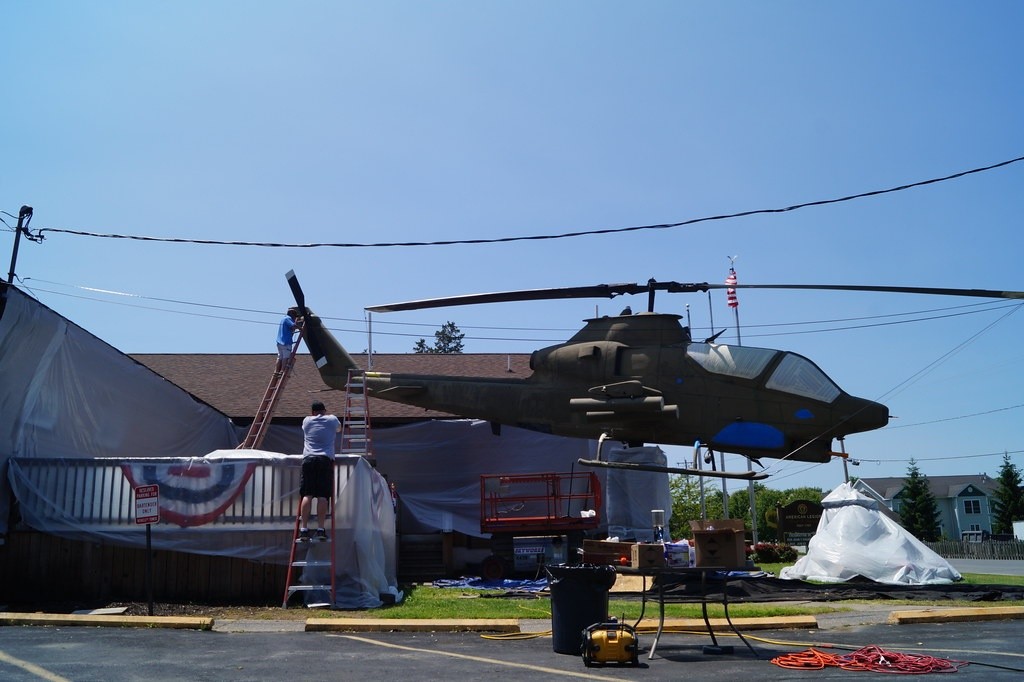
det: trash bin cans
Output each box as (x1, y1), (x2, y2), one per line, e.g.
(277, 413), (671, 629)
(547, 562), (617, 655)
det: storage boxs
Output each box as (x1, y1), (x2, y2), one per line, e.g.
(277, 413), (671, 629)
(688, 519), (748, 568)
(581, 540), (695, 570)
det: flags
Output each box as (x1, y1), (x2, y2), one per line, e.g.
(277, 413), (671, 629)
(724, 269), (739, 308)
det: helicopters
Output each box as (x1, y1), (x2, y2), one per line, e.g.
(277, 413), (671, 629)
(284, 268), (1024, 481)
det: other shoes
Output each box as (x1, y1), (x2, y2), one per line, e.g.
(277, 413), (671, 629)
(278, 372), (290, 378)
(272, 372), (278, 378)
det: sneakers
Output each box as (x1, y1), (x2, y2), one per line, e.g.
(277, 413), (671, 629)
(317, 528), (329, 540)
(299, 528), (312, 540)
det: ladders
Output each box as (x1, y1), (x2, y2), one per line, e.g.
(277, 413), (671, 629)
(341, 369), (375, 456)
(282, 464), (337, 611)
(241, 323), (305, 449)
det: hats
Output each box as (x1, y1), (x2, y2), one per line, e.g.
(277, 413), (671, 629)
(312, 402), (325, 411)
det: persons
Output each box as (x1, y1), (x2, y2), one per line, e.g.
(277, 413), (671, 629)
(299, 402), (342, 541)
(272, 309), (305, 378)
